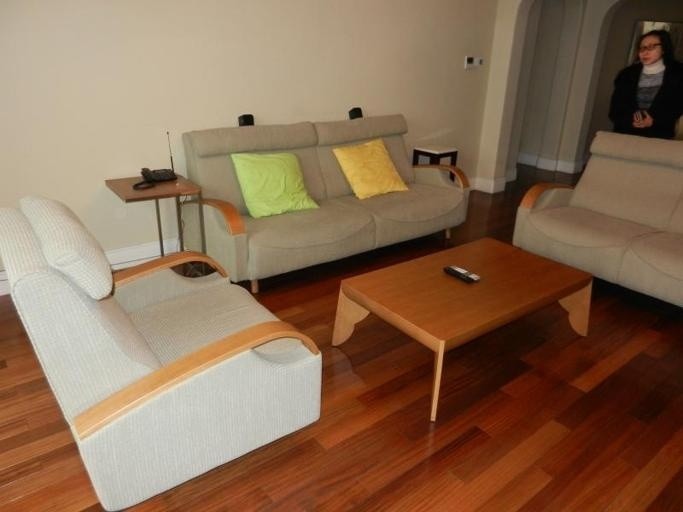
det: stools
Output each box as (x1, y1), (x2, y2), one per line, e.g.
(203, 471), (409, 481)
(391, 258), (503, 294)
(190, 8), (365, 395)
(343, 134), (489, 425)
(413, 146), (458, 183)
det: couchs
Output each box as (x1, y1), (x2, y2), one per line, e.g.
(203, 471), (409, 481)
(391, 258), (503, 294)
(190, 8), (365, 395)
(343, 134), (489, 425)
(183, 113), (468, 293)
(513, 129), (683, 309)
(4, 194), (324, 508)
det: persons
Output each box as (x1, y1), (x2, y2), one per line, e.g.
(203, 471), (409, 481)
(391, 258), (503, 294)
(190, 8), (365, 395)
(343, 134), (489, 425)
(609, 29), (681, 138)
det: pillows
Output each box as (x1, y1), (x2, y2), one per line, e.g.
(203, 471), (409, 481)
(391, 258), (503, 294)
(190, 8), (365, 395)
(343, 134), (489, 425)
(229, 151), (322, 220)
(333, 138), (411, 202)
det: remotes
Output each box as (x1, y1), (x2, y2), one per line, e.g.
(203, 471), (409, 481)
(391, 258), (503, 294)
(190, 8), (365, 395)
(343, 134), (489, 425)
(445, 266), (480, 281)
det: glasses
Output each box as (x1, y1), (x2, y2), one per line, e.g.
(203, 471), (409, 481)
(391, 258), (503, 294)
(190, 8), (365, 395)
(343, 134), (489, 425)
(640, 43), (661, 53)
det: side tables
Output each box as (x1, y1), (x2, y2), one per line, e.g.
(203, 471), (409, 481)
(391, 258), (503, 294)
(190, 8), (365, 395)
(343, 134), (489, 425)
(102, 169), (207, 260)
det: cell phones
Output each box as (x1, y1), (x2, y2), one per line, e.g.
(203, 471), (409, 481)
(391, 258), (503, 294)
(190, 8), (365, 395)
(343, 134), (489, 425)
(637, 110), (647, 121)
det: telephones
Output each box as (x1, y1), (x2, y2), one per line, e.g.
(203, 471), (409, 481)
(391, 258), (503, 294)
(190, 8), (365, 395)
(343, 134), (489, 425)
(141, 168), (177, 183)
